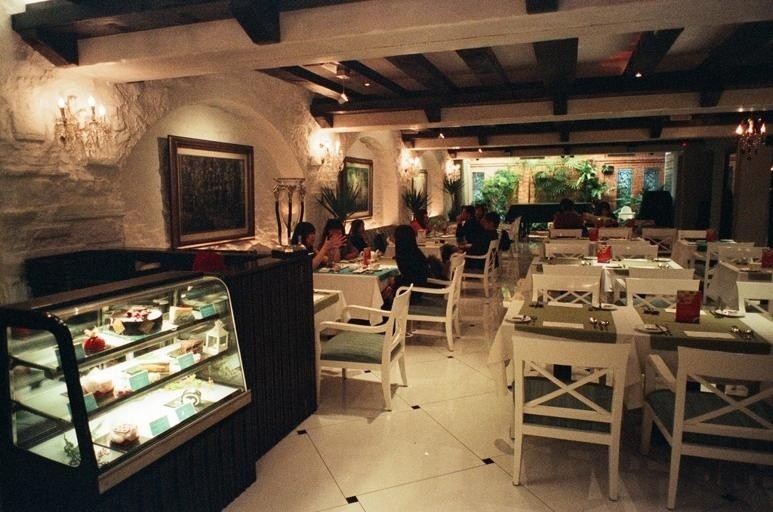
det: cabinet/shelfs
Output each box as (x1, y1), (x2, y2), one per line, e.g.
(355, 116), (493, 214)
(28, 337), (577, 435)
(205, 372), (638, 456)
(0, 269), (257, 512)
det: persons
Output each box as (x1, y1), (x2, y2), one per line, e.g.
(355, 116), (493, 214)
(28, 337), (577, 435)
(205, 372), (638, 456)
(318, 219), (359, 263)
(378, 224), (427, 310)
(553, 198), (579, 229)
(290, 220), (346, 272)
(441, 203), (501, 266)
(592, 200), (616, 227)
(348, 219), (370, 252)
(409, 209), (431, 233)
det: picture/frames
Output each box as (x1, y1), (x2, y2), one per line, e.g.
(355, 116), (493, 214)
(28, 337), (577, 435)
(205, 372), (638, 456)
(336, 156), (373, 222)
(412, 169), (428, 214)
(167, 135), (255, 251)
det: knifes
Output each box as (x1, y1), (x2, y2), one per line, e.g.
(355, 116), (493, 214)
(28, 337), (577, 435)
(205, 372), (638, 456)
(654, 323), (665, 334)
(598, 302), (601, 310)
(708, 310), (715, 316)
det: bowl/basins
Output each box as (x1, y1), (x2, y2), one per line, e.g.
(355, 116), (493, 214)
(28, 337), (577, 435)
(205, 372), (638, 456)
(746, 262), (762, 272)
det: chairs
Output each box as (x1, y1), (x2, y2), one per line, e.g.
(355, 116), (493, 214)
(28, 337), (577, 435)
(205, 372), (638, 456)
(312, 280), (413, 412)
(397, 261), (465, 350)
(641, 343), (772, 510)
(452, 239), (499, 297)
(486, 216), (773, 417)
(510, 333), (633, 500)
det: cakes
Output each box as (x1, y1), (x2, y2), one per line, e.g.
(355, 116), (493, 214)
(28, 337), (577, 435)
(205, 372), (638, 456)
(181, 390), (202, 407)
(110, 425), (140, 451)
(81, 328), (106, 354)
(108, 305), (163, 335)
(169, 306), (195, 325)
(141, 361), (169, 376)
(84, 374), (114, 400)
(181, 339), (203, 354)
(153, 296), (169, 313)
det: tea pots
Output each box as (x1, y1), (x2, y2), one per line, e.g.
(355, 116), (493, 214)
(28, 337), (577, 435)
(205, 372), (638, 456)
(359, 249), (379, 262)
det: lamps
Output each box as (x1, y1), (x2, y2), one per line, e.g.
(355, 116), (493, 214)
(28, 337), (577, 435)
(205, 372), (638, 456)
(734, 112), (768, 162)
(272, 177), (307, 247)
(337, 79), (348, 105)
(335, 61), (351, 80)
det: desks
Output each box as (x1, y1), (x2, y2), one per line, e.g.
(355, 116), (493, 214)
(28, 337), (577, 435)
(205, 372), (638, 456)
(312, 216), (471, 329)
(506, 202), (592, 237)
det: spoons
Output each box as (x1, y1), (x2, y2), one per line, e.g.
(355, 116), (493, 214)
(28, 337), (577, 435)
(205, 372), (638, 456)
(589, 314), (597, 331)
(730, 323), (746, 341)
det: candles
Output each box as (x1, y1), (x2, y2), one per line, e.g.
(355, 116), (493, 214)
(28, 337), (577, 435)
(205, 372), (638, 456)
(335, 141), (340, 154)
(58, 97), (66, 116)
(89, 97), (96, 115)
(97, 105), (107, 119)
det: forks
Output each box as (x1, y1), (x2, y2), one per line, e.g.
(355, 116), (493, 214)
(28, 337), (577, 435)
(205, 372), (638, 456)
(643, 306), (658, 314)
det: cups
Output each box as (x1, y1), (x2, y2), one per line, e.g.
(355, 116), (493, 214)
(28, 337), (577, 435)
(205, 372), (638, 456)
(439, 239), (444, 245)
(363, 248), (370, 265)
(434, 239), (438, 245)
(332, 263), (341, 273)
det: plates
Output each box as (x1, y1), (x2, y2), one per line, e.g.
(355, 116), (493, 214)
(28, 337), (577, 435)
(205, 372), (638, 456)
(352, 269), (366, 274)
(504, 314), (531, 323)
(741, 267), (750, 271)
(328, 264), (349, 271)
(714, 308), (744, 317)
(633, 323), (668, 334)
(583, 256), (597, 261)
(606, 263), (621, 268)
(654, 258), (671, 263)
(593, 302), (617, 310)
